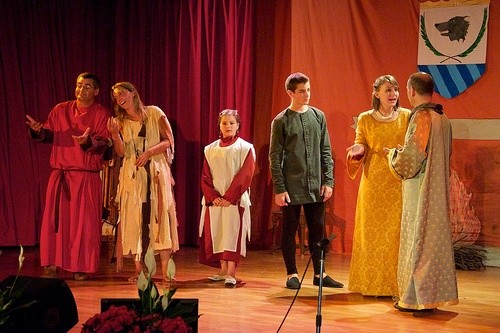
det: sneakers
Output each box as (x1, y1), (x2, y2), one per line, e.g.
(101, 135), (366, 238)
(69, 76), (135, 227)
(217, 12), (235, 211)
(40, 264), (57, 278)
(74, 272), (87, 280)
(286, 277), (301, 289)
(313, 274), (343, 288)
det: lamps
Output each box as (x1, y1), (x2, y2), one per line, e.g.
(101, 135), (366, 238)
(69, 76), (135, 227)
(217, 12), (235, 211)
(0, 275), (79, 333)
(100, 298), (198, 333)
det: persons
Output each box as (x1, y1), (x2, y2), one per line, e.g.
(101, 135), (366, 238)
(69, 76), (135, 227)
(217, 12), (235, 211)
(107, 83), (180, 287)
(25, 72), (115, 281)
(346, 75), (412, 298)
(269, 72), (343, 289)
(199, 110), (256, 285)
(384, 72), (460, 312)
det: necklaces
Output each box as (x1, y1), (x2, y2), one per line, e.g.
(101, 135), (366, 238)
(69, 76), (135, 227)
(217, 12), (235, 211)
(376, 108), (395, 120)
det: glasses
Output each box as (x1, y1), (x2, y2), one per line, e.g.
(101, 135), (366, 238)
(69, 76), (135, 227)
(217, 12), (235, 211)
(76, 83), (94, 90)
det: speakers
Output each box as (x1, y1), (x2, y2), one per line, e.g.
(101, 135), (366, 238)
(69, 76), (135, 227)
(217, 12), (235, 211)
(0, 275), (79, 333)
(101, 298), (198, 333)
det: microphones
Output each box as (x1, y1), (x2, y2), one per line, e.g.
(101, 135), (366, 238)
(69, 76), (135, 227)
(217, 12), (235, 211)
(316, 233), (337, 248)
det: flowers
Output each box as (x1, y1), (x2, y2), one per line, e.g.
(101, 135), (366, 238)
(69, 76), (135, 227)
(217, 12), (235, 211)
(449, 166), (489, 272)
(81, 240), (204, 333)
(0, 244), (38, 333)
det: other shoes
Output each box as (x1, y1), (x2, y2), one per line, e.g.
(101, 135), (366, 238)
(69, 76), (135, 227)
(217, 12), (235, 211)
(128, 275), (138, 282)
(224, 275), (236, 285)
(163, 277), (176, 282)
(393, 302), (433, 312)
(392, 295), (400, 301)
(208, 275), (226, 280)
